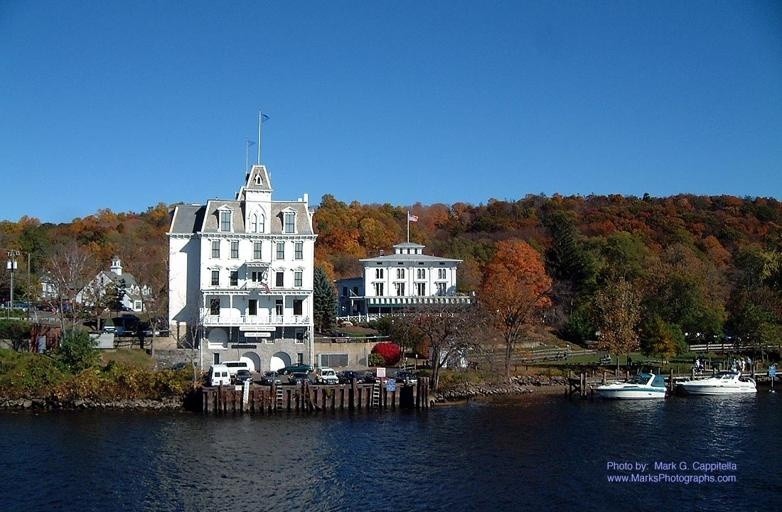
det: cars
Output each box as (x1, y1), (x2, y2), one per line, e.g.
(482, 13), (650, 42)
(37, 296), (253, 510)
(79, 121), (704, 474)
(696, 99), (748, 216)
(321, 319), (378, 343)
(232, 362), (418, 387)
(1, 296), (164, 337)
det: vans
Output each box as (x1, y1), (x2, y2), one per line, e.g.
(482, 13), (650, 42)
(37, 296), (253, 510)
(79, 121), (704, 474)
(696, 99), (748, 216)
(220, 361), (253, 377)
(205, 364), (232, 386)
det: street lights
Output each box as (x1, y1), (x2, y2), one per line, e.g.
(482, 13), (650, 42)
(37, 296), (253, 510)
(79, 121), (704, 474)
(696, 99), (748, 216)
(5, 249), (21, 311)
(683, 332), (733, 355)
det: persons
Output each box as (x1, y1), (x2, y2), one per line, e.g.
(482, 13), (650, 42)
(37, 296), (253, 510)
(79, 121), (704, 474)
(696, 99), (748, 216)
(692, 355), (778, 379)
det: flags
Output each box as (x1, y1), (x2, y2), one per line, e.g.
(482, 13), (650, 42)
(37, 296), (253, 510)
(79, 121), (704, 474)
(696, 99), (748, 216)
(409, 212), (419, 221)
(261, 113), (271, 123)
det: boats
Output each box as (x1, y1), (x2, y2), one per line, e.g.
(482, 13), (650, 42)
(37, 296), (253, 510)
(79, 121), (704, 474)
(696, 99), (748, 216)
(592, 371), (669, 401)
(673, 367), (759, 397)
